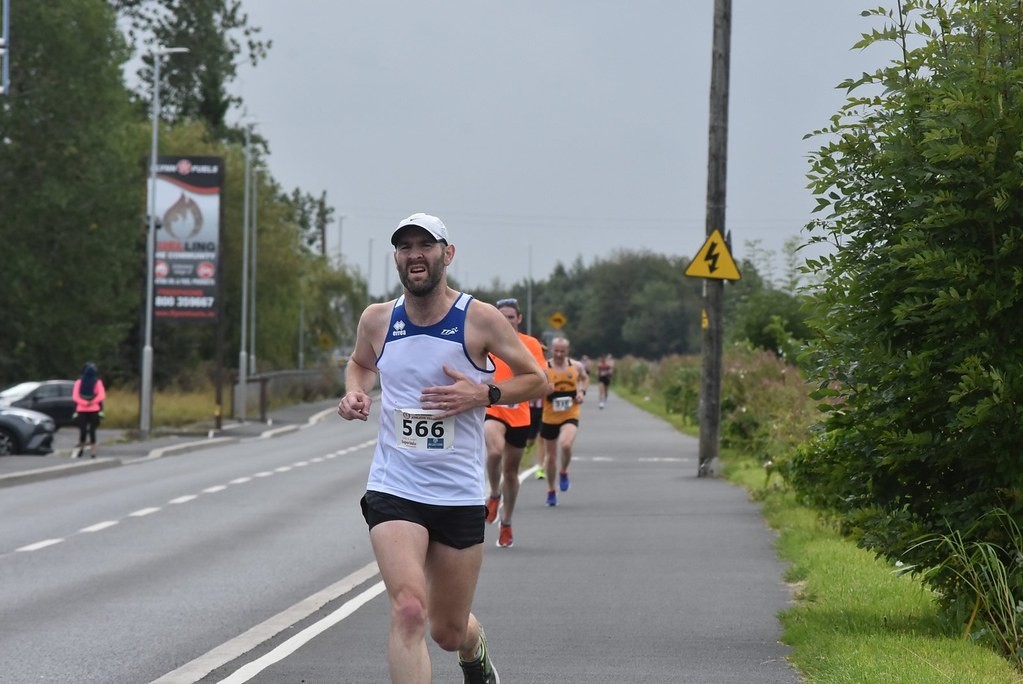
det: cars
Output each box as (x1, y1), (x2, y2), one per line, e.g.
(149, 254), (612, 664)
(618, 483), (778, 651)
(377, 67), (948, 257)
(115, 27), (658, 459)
(0, 407), (56, 456)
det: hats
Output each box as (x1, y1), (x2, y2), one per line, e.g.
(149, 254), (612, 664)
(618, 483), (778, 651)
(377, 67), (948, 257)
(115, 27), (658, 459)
(391, 213), (449, 248)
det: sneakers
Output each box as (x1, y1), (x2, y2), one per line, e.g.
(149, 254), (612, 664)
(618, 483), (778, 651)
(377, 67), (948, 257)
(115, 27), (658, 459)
(485, 494), (503, 523)
(457, 629), (500, 684)
(559, 472), (569, 491)
(546, 492), (557, 505)
(537, 470), (544, 478)
(497, 522), (513, 547)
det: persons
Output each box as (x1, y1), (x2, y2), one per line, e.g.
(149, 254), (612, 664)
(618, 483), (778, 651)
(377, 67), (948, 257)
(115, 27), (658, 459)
(479, 299), (554, 549)
(542, 337), (589, 507)
(339, 212), (548, 684)
(73, 364), (106, 458)
(525, 398), (546, 480)
(581, 355), (592, 374)
(598, 353), (614, 409)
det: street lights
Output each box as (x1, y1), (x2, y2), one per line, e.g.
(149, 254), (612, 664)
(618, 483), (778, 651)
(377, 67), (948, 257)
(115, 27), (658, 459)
(139, 48), (190, 431)
(338, 214), (389, 307)
(236, 120), (260, 422)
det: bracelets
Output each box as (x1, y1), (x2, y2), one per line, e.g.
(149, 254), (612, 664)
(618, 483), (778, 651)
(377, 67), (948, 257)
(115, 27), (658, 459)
(581, 389), (585, 395)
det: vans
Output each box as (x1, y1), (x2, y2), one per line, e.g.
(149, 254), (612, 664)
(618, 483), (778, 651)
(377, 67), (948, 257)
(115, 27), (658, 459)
(0, 380), (103, 433)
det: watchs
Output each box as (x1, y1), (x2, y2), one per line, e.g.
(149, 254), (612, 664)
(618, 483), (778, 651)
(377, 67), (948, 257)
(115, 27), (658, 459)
(487, 384), (501, 409)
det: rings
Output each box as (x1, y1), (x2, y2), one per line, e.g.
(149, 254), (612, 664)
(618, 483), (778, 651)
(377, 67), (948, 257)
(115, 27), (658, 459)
(444, 402), (447, 408)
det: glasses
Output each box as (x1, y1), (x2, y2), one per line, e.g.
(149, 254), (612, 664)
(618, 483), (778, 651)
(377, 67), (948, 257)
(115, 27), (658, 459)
(497, 298), (519, 309)
